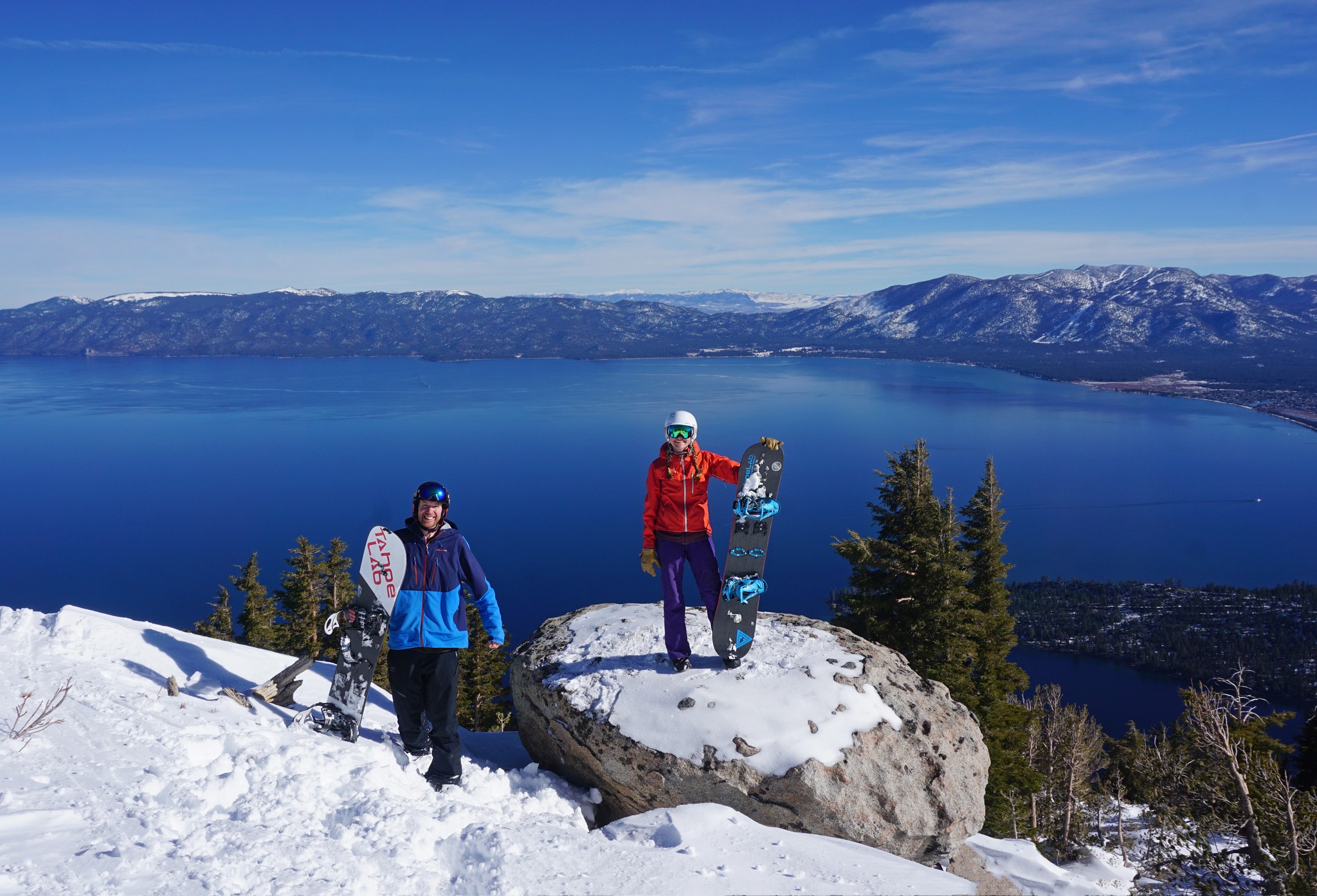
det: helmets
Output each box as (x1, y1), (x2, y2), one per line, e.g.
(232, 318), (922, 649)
(412, 481), (450, 524)
(664, 411), (698, 443)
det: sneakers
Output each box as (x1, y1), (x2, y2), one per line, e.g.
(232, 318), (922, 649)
(673, 657), (690, 672)
(404, 744), (430, 756)
(424, 771), (461, 785)
(723, 658), (741, 669)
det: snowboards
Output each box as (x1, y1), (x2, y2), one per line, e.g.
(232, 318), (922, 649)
(711, 442), (785, 660)
(326, 527), (407, 744)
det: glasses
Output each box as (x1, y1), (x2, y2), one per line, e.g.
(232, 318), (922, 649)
(415, 482), (449, 503)
(667, 424), (693, 439)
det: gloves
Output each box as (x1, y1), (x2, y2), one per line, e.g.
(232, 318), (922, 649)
(641, 549), (661, 577)
(760, 437), (784, 451)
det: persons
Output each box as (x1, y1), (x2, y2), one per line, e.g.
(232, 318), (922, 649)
(640, 410), (783, 672)
(342, 482), (504, 793)
